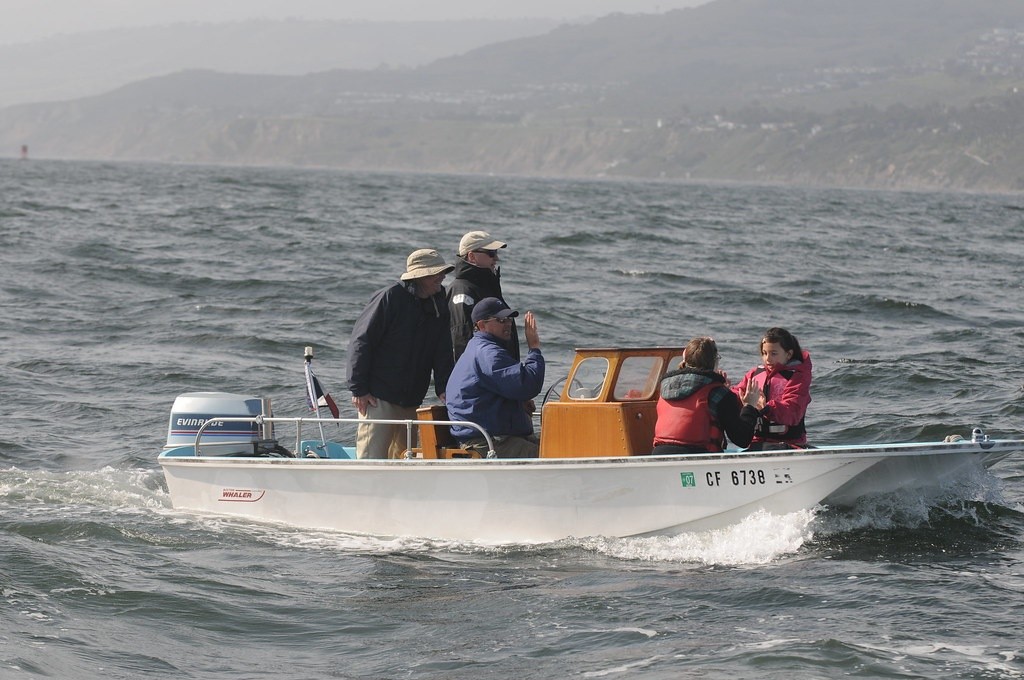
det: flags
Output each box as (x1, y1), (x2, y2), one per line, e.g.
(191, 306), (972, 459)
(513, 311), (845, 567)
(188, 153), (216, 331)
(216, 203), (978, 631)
(307, 371), (339, 428)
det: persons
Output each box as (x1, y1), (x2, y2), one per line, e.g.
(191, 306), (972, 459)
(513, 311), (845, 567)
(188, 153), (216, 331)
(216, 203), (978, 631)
(653, 336), (761, 455)
(446, 230), (520, 365)
(446, 297), (545, 458)
(346, 249), (454, 459)
(718, 327), (813, 451)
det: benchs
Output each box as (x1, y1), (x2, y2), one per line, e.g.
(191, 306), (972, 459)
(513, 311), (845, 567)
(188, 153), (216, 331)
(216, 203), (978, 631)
(399, 404), (487, 458)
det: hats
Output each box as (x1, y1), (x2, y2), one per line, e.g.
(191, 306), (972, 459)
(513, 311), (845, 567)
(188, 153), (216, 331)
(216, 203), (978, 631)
(459, 231), (507, 256)
(400, 249), (456, 281)
(471, 297), (519, 323)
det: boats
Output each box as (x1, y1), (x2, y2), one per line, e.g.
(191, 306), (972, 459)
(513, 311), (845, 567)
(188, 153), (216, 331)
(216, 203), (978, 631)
(158, 389), (1024, 547)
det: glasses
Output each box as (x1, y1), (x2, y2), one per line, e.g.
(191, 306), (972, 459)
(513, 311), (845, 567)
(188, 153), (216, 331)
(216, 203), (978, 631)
(716, 354), (721, 360)
(484, 317), (513, 323)
(474, 250), (497, 257)
